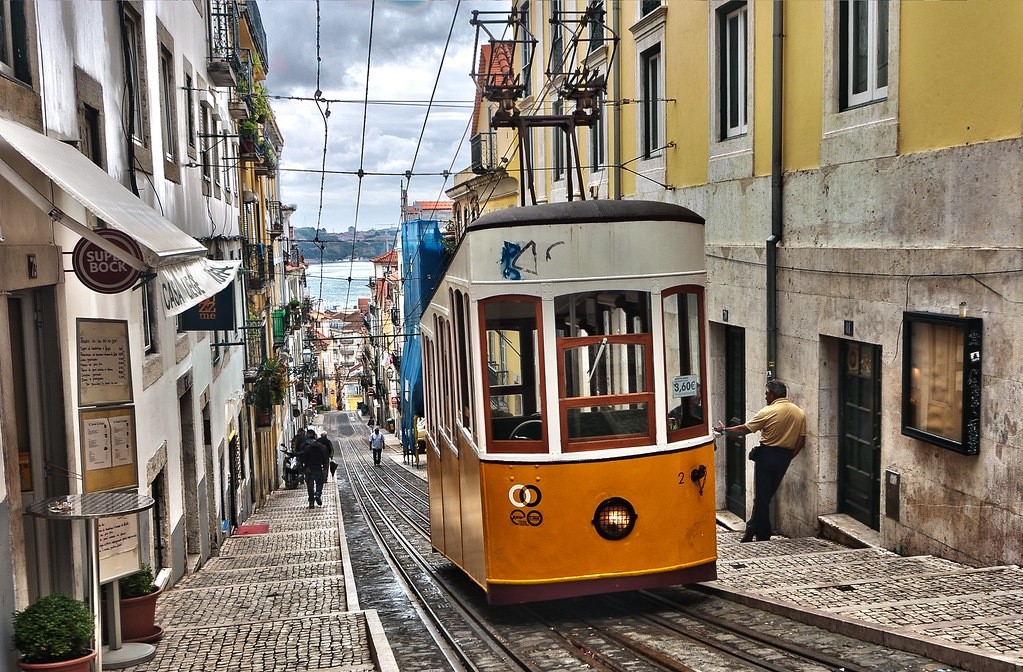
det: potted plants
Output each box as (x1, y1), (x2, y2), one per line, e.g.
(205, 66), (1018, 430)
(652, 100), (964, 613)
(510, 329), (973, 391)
(289, 299), (301, 312)
(11, 592), (98, 672)
(101, 562), (164, 644)
(247, 357), (285, 427)
(239, 121), (256, 153)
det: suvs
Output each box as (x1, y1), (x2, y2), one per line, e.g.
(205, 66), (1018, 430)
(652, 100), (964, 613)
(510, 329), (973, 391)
(399, 418), (427, 454)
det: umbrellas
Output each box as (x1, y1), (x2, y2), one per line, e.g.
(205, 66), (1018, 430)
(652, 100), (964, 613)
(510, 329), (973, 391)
(330, 457), (339, 477)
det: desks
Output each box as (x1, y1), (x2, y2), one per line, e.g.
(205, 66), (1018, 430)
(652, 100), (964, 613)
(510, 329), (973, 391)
(27, 492), (155, 671)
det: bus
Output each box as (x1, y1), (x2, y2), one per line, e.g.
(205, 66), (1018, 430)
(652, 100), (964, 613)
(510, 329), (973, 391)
(419, 1), (723, 606)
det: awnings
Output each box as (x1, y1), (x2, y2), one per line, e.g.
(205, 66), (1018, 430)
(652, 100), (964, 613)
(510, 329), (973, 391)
(0, 115), (243, 319)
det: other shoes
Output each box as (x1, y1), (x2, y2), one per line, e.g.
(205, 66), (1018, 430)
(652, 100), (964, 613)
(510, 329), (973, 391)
(741, 534), (753, 541)
(323, 480), (328, 483)
(377, 460), (380, 464)
(756, 534), (770, 541)
(315, 496), (322, 505)
(374, 462), (377, 466)
(309, 501), (314, 508)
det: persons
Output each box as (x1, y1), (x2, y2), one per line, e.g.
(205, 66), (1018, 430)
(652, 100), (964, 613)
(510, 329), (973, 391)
(369, 428), (386, 466)
(713, 379), (806, 543)
(669, 384), (701, 429)
(367, 416), (374, 433)
(291, 428), (334, 508)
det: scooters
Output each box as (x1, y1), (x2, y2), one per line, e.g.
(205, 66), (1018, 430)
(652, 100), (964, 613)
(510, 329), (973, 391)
(279, 443), (300, 489)
